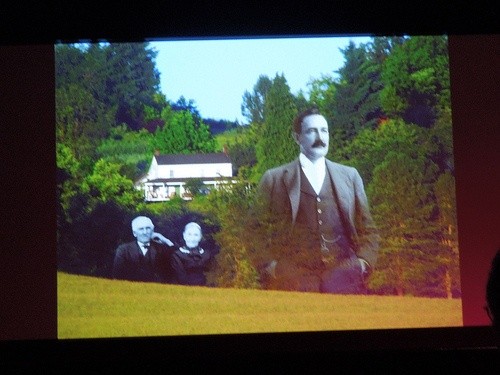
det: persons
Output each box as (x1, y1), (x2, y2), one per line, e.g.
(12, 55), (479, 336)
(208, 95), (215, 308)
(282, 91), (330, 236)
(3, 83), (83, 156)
(114, 216), (179, 283)
(172, 222), (212, 286)
(242, 111), (380, 295)
